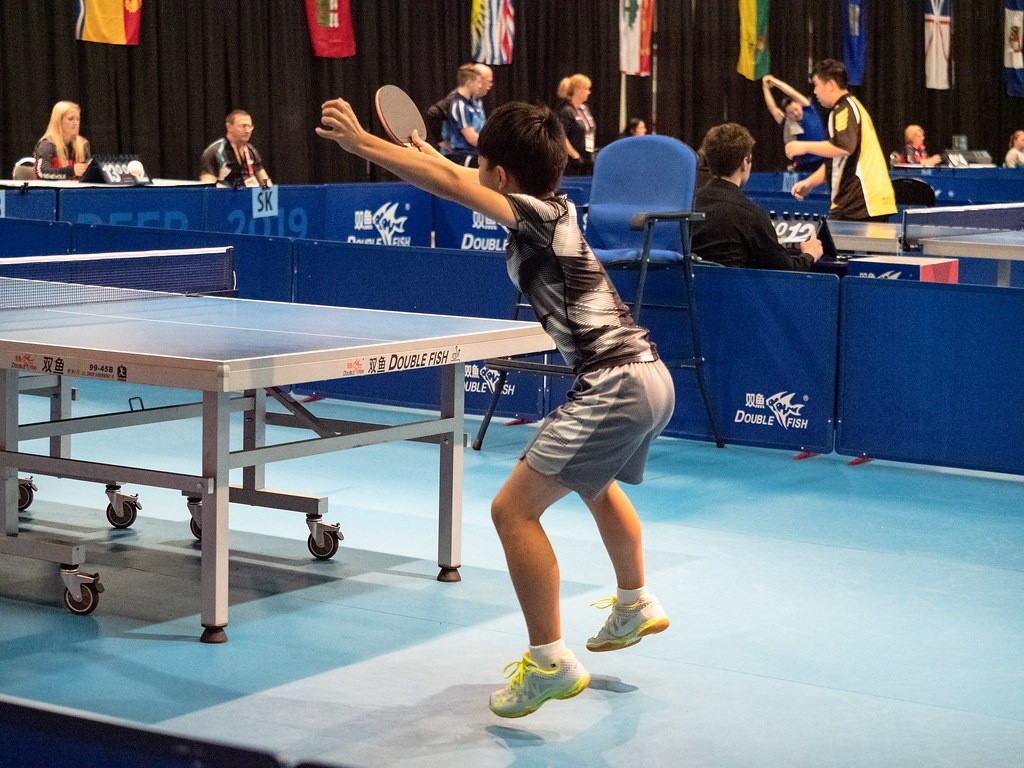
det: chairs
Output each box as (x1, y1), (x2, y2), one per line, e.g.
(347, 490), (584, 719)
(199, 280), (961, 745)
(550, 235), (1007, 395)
(892, 178), (937, 209)
(469, 134), (727, 450)
(12, 156), (35, 180)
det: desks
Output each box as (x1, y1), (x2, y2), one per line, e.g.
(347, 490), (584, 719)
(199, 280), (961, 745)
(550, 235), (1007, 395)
(0, 244), (559, 648)
(826, 202), (1024, 288)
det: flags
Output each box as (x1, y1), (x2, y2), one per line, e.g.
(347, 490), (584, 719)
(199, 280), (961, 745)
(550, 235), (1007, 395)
(924, 0), (951, 89)
(75, 0), (141, 46)
(619, 0), (654, 77)
(1003, 0), (1024, 97)
(842, 0), (867, 85)
(471, 0), (514, 64)
(737, 1), (770, 81)
(305, 0), (356, 57)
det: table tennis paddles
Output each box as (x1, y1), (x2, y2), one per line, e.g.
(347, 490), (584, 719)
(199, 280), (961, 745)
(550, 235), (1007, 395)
(376, 85), (428, 151)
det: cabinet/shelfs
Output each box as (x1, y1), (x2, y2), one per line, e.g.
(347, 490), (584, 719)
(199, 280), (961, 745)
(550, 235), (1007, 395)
(0, 177), (217, 190)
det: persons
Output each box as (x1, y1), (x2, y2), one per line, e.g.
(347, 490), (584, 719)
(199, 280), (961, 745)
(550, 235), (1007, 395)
(619, 118), (646, 141)
(198, 109), (273, 187)
(901, 125), (942, 166)
(696, 148), (711, 189)
(425, 62), (493, 169)
(786, 58), (899, 222)
(692, 123), (823, 272)
(558, 74), (595, 176)
(447, 65), (483, 169)
(315, 96), (675, 719)
(1005, 131), (1024, 171)
(763, 74), (827, 171)
(34, 101), (93, 181)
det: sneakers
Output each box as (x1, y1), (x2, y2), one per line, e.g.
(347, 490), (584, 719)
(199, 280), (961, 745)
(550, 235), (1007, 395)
(490, 649), (591, 719)
(586, 594), (670, 651)
